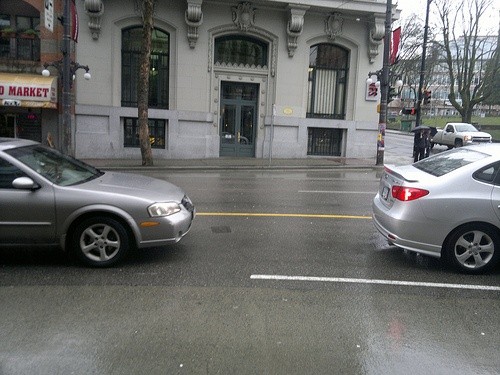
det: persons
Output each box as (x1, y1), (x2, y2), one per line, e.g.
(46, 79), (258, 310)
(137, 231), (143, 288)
(414, 129), (434, 163)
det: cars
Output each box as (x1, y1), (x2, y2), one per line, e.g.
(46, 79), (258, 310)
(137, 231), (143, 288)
(0, 136), (195, 268)
(371, 142), (500, 274)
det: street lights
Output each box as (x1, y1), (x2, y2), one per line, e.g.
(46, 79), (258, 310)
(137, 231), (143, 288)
(42, 56), (92, 156)
(369, 68), (403, 167)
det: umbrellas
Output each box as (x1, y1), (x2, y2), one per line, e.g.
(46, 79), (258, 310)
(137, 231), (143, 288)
(411, 126), (437, 137)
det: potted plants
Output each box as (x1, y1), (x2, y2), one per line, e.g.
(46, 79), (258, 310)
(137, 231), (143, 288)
(20, 29), (35, 39)
(0, 25), (16, 38)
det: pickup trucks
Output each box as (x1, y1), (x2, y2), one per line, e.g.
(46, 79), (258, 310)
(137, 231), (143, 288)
(428, 121), (492, 149)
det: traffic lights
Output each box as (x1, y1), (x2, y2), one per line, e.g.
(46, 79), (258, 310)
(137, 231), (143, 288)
(402, 107), (416, 115)
(424, 91), (431, 105)
